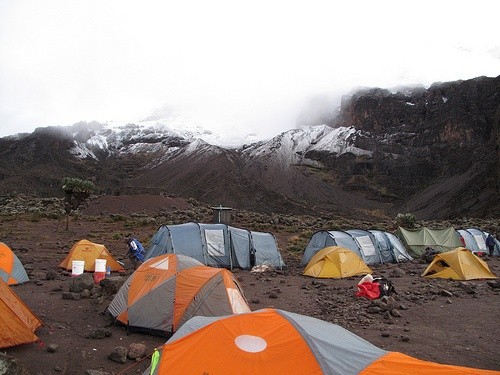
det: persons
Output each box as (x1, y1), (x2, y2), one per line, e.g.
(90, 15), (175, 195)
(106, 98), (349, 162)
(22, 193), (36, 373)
(486, 234), (496, 257)
(124, 236), (145, 270)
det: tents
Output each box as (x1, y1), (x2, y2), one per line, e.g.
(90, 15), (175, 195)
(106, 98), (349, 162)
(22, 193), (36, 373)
(421, 247), (499, 281)
(395, 226), (465, 258)
(0, 277), (43, 348)
(0, 242), (30, 285)
(59, 240), (124, 271)
(139, 307), (500, 375)
(107, 253), (251, 338)
(457, 229), (500, 255)
(144, 221), (286, 270)
(302, 246), (373, 278)
(302, 228), (414, 266)
